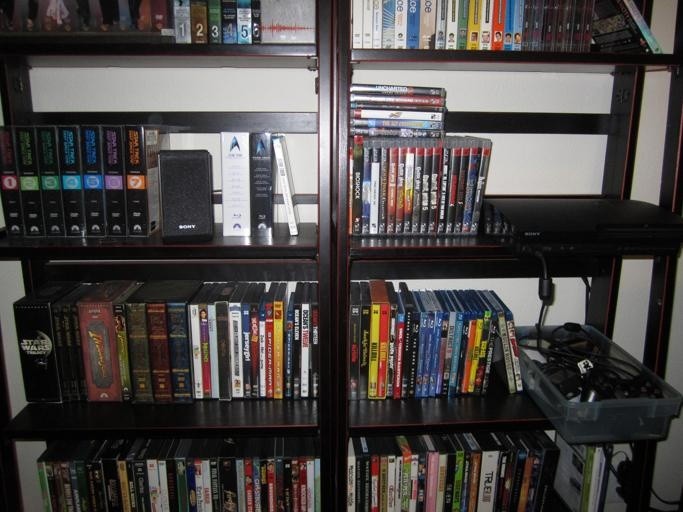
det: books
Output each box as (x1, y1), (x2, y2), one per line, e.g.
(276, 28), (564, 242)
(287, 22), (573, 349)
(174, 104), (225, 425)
(2, 126), (298, 237)
(35, 433), (321, 511)
(348, 83), (491, 238)
(351, 0), (664, 56)
(13, 279), (318, 404)
(346, 430), (606, 511)
(348, 280), (524, 402)
(2, 2), (262, 44)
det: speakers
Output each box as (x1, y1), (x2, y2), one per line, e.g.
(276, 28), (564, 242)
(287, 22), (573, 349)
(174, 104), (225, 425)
(158, 149), (215, 244)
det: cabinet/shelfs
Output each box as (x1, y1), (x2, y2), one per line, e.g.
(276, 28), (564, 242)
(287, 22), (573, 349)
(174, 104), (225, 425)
(0, 0), (333, 512)
(335, 0), (683, 512)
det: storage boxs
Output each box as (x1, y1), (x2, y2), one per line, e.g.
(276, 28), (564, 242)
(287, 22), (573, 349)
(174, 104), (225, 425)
(516, 325), (682, 444)
